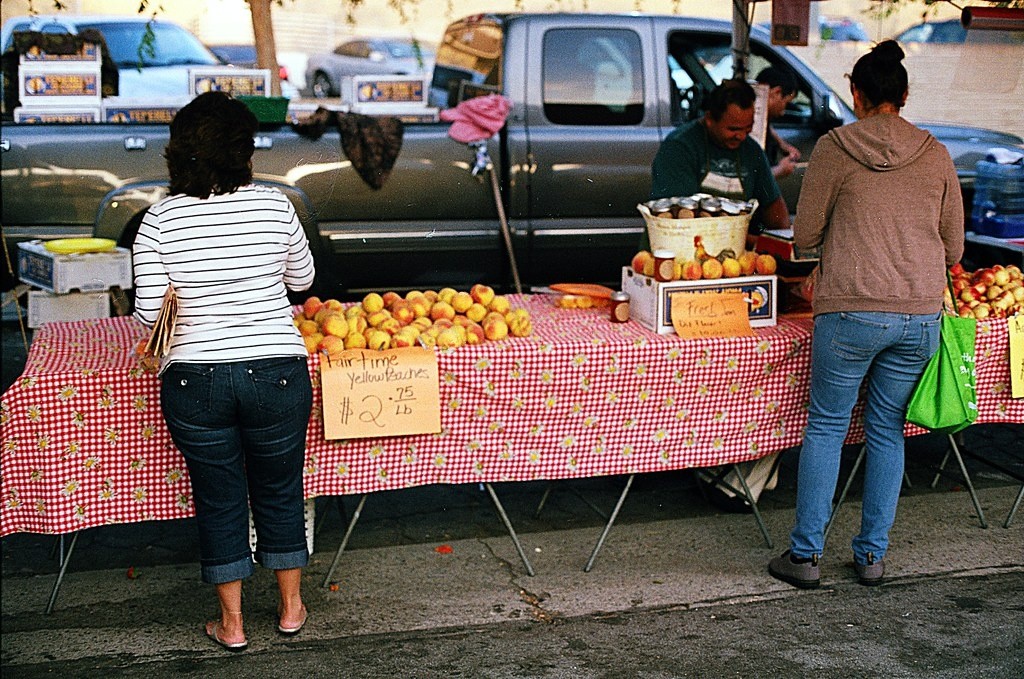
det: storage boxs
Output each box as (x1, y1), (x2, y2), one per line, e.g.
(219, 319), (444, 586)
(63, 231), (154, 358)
(16, 238), (133, 331)
(14, 30), (439, 130)
(621, 266), (778, 334)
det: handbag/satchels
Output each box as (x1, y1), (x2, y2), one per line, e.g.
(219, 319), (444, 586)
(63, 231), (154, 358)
(913, 270), (978, 434)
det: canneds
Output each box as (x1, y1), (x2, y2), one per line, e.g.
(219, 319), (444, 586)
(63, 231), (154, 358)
(611, 291), (631, 322)
(645, 192), (752, 219)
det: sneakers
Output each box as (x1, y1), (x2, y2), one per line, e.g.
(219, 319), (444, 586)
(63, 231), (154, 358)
(770, 549), (820, 589)
(855, 552), (884, 585)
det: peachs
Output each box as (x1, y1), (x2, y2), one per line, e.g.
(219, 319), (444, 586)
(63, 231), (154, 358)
(945, 261), (1024, 317)
(632, 250), (776, 281)
(294, 283), (530, 355)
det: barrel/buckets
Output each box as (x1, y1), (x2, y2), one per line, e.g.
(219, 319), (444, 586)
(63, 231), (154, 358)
(638, 199), (759, 259)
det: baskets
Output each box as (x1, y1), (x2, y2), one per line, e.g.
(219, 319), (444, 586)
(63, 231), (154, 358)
(637, 198), (760, 259)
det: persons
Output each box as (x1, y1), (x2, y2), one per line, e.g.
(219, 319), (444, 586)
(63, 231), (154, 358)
(131, 91), (315, 650)
(767, 38), (965, 590)
(751, 66), (800, 178)
(637, 78), (788, 255)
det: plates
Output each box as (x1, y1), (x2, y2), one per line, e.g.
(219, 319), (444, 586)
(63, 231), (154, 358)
(44, 237), (116, 255)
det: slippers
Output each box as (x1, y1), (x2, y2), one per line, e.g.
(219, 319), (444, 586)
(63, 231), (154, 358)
(205, 619), (248, 653)
(277, 601), (309, 633)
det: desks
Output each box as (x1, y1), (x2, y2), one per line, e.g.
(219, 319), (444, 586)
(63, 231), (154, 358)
(0, 285), (1024, 615)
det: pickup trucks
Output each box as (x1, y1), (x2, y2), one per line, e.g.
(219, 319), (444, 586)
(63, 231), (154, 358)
(0, 0), (1024, 306)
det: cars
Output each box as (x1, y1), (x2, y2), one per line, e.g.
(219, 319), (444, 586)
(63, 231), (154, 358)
(304, 37), (438, 97)
(1, 16), (302, 102)
(758, 13), (964, 44)
